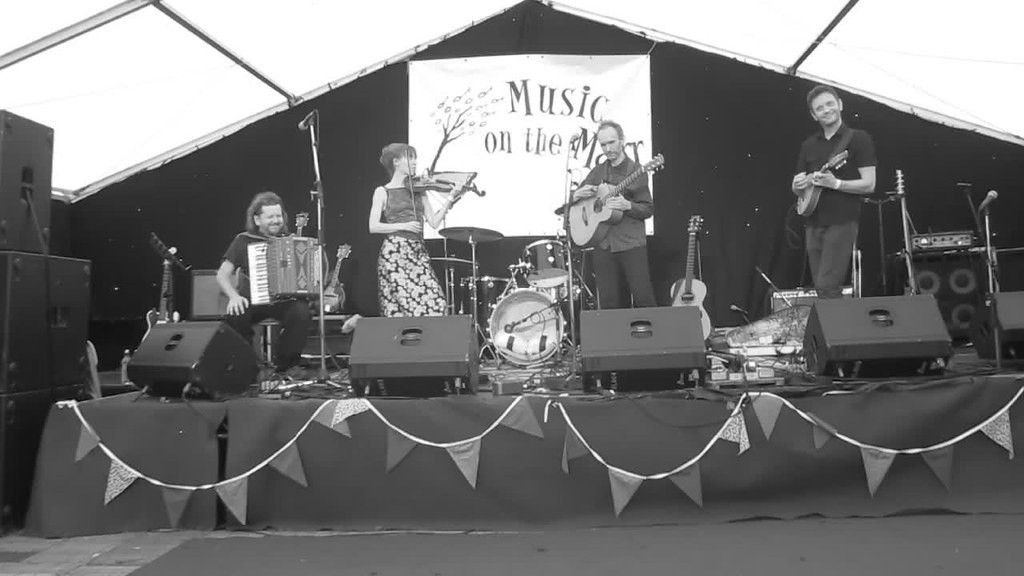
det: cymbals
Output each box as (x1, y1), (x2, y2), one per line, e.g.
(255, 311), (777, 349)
(439, 226), (505, 242)
(431, 256), (479, 268)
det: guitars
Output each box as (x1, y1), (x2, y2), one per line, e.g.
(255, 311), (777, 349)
(567, 152), (665, 250)
(668, 214), (712, 342)
(796, 149), (848, 217)
(140, 245), (180, 341)
(316, 243), (352, 312)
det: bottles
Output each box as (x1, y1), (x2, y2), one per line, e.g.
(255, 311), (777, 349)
(121, 349), (132, 384)
(341, 314), (362, 333)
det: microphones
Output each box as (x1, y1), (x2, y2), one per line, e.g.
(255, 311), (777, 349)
(298, 108), (319, 131)
(977, 189), (999, 216)
(569, 132), (583, 144)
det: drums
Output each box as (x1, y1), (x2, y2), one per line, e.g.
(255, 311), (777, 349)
(488, 288), (566, 367)
(461, 276), (511, 332)
(524, 239), (570, 289)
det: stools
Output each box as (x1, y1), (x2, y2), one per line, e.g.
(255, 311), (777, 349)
(257, 318), (280, 362)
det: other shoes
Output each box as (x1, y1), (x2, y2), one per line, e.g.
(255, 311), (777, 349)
(286, 366), (310, 380)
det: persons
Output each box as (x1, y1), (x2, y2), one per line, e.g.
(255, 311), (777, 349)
(369, 143), (463, 317)
(216, 192), (312, 381)
(792, 85), (877, 298)
(568, 120), (657, 309)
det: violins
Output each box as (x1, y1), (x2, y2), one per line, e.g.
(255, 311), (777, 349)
(404, 175), (487, 198)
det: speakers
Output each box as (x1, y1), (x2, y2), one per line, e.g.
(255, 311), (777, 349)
(1, 111), (55, 255)
(125, 321), (264, 398)
(971, 290), (1024, 358)
(2, 385), (91, 521)
(348, 314), (481, 394)
(896, 248), (995, 339)
(0, 251), (94, 395)
(579, 306), (706, 389)
(802, 293), (952, 378)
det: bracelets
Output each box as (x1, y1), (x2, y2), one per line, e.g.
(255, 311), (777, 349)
(447, 195), (454, 203)
(834, 179), (841, 190)
